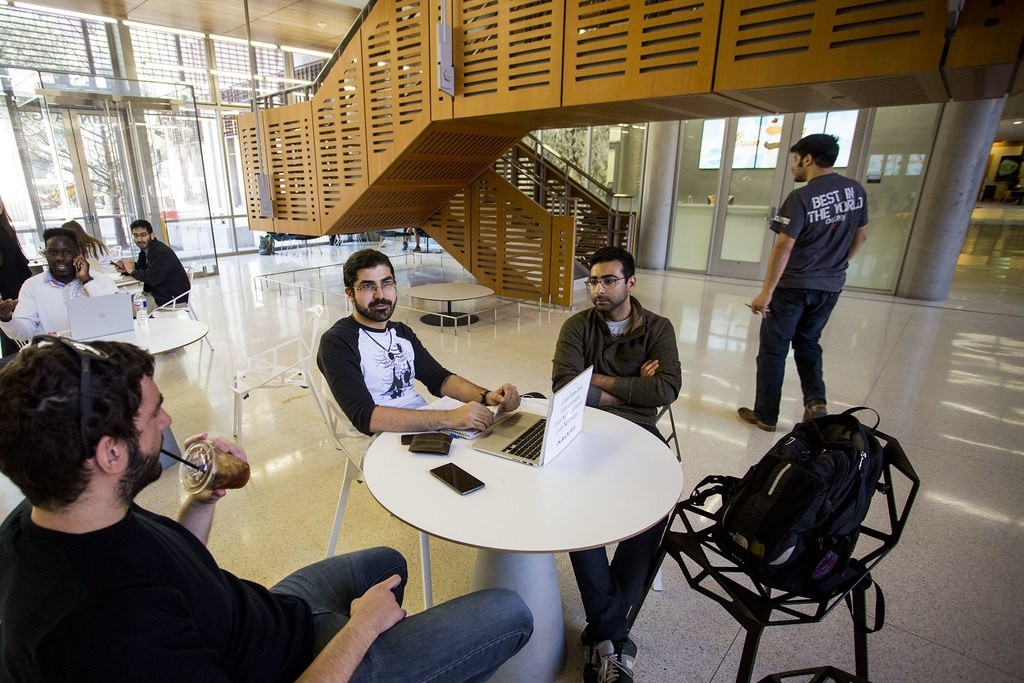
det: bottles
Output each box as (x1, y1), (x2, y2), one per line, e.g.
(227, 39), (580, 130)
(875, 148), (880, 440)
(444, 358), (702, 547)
(133, 289), (151, 331)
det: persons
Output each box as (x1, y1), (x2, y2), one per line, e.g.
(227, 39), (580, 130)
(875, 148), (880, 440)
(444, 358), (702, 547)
(0, 228), (118, 341)
(551, 247), (682, 683)
(0, 341), (534, 683)
(0, 195), (33, 357)
(62, 220), (110, 263)
(738, 134), (868, 431)
(317, 248), (521, 437)
(115, 220), (191, 318)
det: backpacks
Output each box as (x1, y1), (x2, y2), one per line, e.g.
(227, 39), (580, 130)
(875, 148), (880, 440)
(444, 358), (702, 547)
(713, 406), (886, 634)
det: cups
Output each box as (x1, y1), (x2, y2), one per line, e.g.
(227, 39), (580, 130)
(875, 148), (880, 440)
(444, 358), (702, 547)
(178, 440), (251, 497)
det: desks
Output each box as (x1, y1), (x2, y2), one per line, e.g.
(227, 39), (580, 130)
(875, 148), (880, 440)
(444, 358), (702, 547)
(18, 316), (211, 469)
(406, 281), (495, 326)
(1002, 188), (1024, 206)
(28, 259), (49, 273)
(112, 273), (140, 295)
(362, 395), (685, 683)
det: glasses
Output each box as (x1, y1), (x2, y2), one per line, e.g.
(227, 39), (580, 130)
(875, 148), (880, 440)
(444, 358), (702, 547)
(30, 333), (108, 454)
(585, 277), (628, 290)
(132, 233), (151, 238)
(351, 278), (398, 295)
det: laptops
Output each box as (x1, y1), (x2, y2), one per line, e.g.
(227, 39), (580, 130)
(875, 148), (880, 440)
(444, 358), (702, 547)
(473, 364), (595, 467)
(60, 291), (134, 341)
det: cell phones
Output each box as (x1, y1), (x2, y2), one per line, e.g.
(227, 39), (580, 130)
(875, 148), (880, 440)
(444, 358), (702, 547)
(430, 463), (485, 496)
(110, 260), (123, 272)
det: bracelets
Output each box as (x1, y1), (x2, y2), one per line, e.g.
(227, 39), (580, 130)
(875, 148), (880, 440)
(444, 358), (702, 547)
(480, 390), (491, 406)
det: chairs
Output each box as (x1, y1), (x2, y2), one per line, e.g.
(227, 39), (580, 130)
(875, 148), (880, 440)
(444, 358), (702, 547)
(321, 373), (434, 612)
(227, 303), (342, 452)
(333, 230), (434, 252)
(625, 423), (921, 683)
(147, 266), (215, 351)
(109, 245), (122, 263)
(652, 361), (682, 593)
(268, 232), (324, 255)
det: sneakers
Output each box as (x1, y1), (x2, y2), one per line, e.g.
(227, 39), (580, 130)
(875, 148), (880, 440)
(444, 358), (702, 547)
(803, 399), (828, 421)
(581, 626), (639, 683)
(738, 407), (776, 434)
